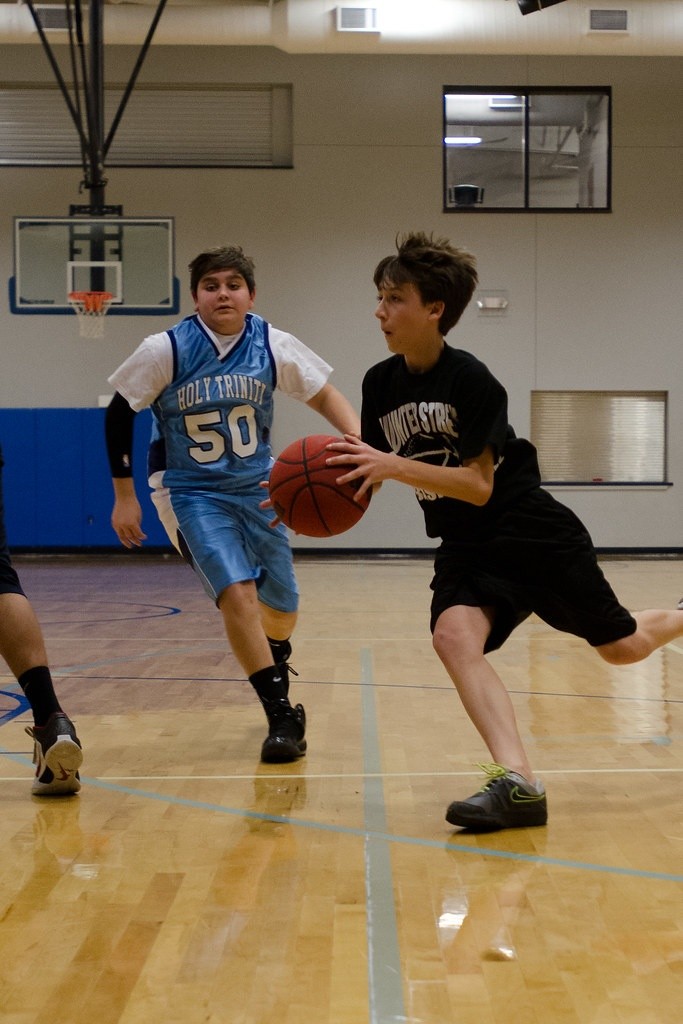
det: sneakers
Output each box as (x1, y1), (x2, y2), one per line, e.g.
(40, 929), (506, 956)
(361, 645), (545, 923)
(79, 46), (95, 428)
(261, 704), (308, 764)
(272, 642), (299, 695)
(445, 761), (549, 829)
(24, 713), (83, 797)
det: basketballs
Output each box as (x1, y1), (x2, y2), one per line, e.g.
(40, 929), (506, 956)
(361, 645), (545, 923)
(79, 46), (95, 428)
(268, 436), (374, 539)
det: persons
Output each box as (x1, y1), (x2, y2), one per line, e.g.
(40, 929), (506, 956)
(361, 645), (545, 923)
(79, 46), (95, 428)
(105, 246), (364, 763)
(258, 232), (683, 827)
(0, 446), (84, 796)
